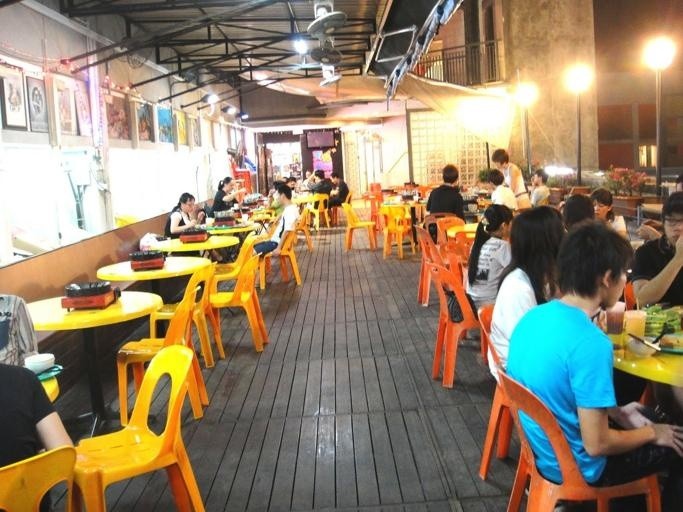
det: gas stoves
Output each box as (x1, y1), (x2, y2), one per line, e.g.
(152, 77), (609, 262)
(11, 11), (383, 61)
(59, 285), (120, 310)
(131, 254), (165, 269)
(179, 232), (207, 242)
(211, 196), (261, 227)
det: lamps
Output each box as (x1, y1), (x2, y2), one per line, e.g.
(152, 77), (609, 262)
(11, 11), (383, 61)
(310, 3), (346, 88)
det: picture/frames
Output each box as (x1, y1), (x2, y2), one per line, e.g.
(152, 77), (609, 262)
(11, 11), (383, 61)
(0, 62), (246, 154)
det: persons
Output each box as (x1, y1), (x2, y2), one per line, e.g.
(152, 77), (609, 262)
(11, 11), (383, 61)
(427, 149), (549, 244)
(165, 167), (349, 257)
(0, 362), (75, 512)
(466, 188), (683, 512)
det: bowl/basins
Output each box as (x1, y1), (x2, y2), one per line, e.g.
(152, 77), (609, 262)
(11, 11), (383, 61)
(23, 352), (54, 373)
(627, 337), (661, 358)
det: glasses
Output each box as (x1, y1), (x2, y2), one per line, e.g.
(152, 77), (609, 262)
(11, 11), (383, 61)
(665, 216), (683, 228)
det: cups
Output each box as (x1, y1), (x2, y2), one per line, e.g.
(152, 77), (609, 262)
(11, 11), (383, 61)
(621, 309), (646, 338)
(604, 301), (625, 335)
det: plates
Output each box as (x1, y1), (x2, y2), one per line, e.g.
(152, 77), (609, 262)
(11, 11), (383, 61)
(31, 363), (61, 381)
(655, 333), (682, 354)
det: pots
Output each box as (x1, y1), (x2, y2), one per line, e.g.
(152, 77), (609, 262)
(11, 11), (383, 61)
(182, 228), (204, 234)
(63, 280), (110, 296)
(127, 250), (161, 260)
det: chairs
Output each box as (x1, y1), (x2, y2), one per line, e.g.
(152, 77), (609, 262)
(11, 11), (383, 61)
(116, 285), (209, 429)
(85, 189), (331, 367)
(362, 169), (682, 309)
(477, 300), (513, 480)
(340, 202), (378, 252)
(69, 344), (206, 512)
(426, 262), (485, 387)
(497, 369), (661, 512)
(0, 445), (77, 511)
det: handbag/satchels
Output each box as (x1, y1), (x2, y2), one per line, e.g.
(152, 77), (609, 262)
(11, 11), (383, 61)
(446, 291), (479, 322)
